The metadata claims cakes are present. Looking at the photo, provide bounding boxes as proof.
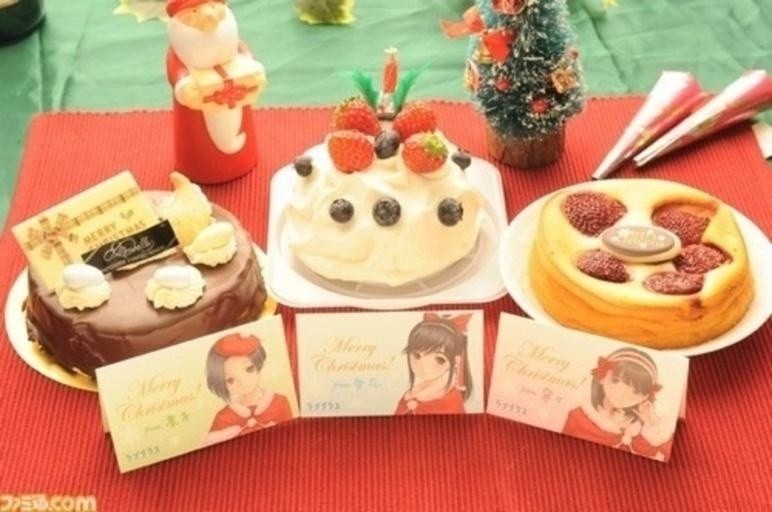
[527,177,754,350]
[286,48,485,286]
[11,171,267,383]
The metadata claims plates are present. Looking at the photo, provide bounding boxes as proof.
[264,152,511,309]
[2,239,277,394]
[497,176,771,356]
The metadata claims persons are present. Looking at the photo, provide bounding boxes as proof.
[560,345,665,459]
[392,311,475,416]
[163,1,267,185]
[205,331,294,445]
